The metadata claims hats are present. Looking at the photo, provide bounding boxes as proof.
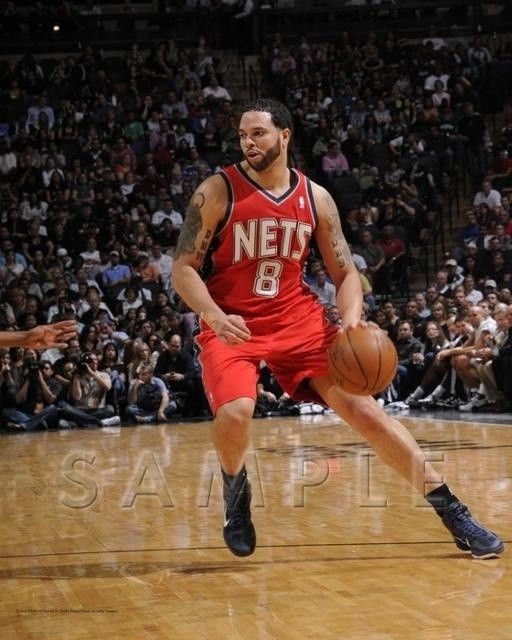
[137,251,149,258]
[110,251,119,256]
[446,260,457,266]
[485,279,497,287]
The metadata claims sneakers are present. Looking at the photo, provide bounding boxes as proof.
[135,414,147,423]
[58,419,77,427]
[39,421,48,428]
[287,404,299,415]
[405,394,510,413]
[7,422,23,431]
[101,416,121,427]
[223,489,256,556]
[443,503,504,558]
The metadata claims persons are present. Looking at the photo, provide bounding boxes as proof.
[1,2,510,435]
[169,98,504,561]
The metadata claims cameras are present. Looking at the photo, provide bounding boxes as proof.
[76,355,92,375]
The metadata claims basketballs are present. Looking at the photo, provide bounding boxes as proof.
[328,325,399,396]
